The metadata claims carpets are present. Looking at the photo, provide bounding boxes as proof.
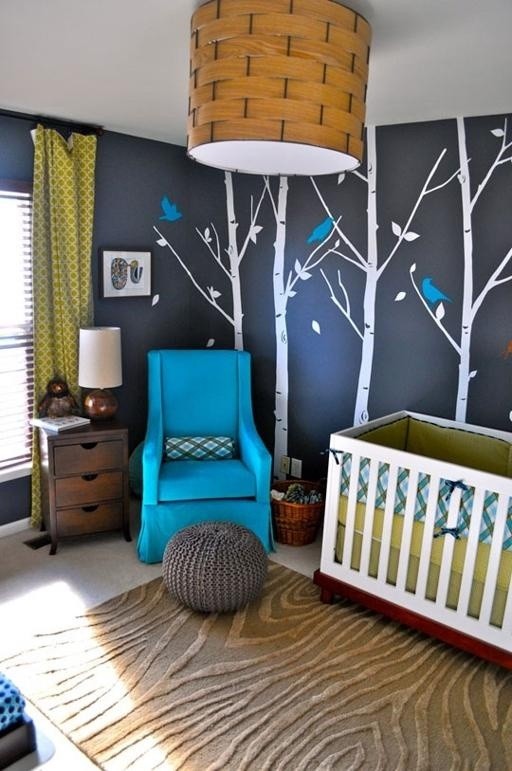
[0,558,512,770]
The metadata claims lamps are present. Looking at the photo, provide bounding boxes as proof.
[78,326,123,421]
[186,0,372,176]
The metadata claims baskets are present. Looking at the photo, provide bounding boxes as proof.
[270,480,326,548]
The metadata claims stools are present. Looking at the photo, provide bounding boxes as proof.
[162,521,269,613]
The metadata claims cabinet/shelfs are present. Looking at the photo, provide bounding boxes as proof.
[38,420,132,555]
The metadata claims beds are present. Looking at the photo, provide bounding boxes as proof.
[313,411,512,671]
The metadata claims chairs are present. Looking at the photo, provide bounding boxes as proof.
[137,349,273,564]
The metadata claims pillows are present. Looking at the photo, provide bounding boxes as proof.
[165,435,237,461]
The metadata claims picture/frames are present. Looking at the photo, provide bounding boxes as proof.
[97,248,155,301]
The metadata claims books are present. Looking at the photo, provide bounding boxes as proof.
[31,414,91,432]
